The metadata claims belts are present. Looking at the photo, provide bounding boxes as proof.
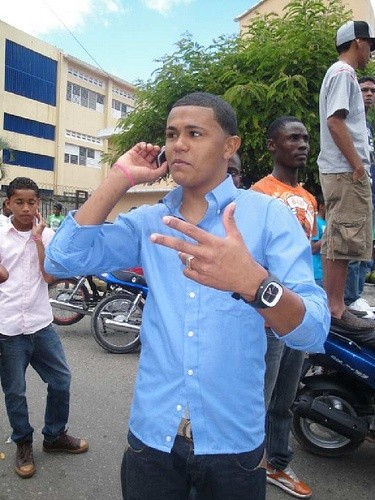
[176,418,193,441]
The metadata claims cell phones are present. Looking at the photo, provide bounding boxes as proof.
[156,149,166,168]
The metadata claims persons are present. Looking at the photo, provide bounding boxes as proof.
[43,93,331,500]
[345,77,375,321]
[320,20,375,333]
[0,199,12,228]
[246,116,318,498]
[224,154,243,187]
[47,203,65,231]
[311,195,327,287]
[0,177,89,478]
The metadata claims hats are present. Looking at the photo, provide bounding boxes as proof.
[335,21,375,52]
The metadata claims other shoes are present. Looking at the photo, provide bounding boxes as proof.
[15,442,36,477]
[331,306,375,330]
[42,436,89,453]
[266,463,313,498]
[347,299,375,320]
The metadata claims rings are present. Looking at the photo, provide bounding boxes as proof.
[186,256,197,269]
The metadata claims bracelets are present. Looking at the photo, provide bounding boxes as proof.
[31,236,42,241]
[111,162,136,187]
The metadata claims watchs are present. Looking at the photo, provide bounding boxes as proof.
[231,263,284,309]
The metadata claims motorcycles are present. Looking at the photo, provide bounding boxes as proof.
[291,321,375,458]
[48,275,136,325]
[91,267,149,353]
